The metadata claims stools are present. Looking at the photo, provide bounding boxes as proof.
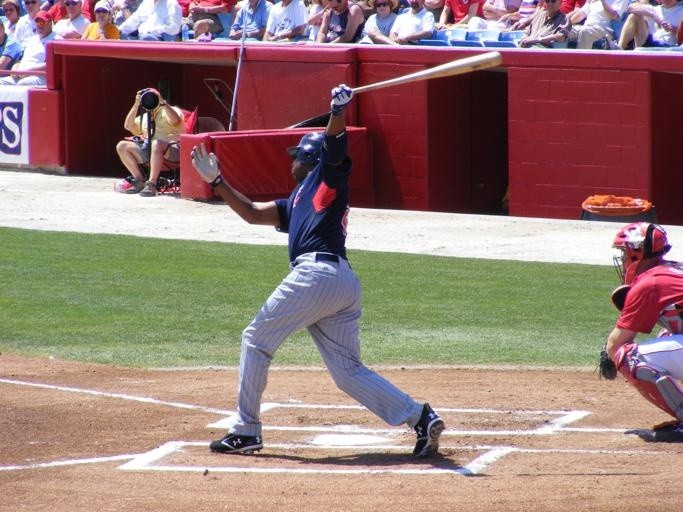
[578,194,660,224]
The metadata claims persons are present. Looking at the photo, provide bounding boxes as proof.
[1,0,683,88]
[596,219,683,440]
[115,88,185,197]
[186,82,445,460]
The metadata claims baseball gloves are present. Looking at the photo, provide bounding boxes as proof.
[611,284,631,311]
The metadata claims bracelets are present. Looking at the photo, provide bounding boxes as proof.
[160,99,168,106]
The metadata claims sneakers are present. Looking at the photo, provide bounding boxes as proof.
[140,180,156,196]
[413,403,444,458]
[653,421,683,433]
[210,435,263,454]
[126,179,143,194]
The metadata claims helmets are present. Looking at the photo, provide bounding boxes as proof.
[612,222,671,285]
[286,133,325,164]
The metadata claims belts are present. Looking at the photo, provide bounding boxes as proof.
[317,254,338,262]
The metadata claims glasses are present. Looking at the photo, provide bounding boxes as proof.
[3,0,110,26]
[375,2,389,7]
[545,0,556,3]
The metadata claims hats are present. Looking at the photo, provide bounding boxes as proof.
[366,0,399,9]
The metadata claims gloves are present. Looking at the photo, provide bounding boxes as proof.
[331,83,353,115]
[191,143,223,186]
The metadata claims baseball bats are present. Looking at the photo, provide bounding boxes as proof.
[349,52,503,93]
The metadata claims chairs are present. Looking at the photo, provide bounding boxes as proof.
[125,107,198,194]
